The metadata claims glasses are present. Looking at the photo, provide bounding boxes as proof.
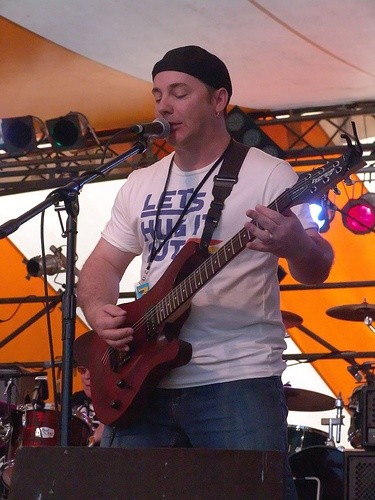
[77,366,88,374]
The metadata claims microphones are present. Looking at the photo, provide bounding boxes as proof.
[128,117,172,137]
[32,380,43,405]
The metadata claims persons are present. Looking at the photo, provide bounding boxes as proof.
[77,46,335,500]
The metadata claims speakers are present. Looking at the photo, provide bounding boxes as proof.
[8,445,300,500]
[343,386,375,500]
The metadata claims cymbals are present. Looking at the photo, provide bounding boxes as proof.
[281,310,304,329]
[326,302,375,322]
[283,386,337,412]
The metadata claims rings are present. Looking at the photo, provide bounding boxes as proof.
[263,233,272,244]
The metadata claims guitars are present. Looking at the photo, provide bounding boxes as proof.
[88,149,369,426]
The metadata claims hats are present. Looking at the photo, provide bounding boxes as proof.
[151,45,233,101]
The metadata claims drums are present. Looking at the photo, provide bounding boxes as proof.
[288,424,345,500]
[0,400,94,490]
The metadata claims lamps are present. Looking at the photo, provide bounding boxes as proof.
[0,116,46,157]
[227,105,289,159]
[342,198,375,235]
[45,112,90,151]
[309,198,335,233]
[23,255,64,280]
